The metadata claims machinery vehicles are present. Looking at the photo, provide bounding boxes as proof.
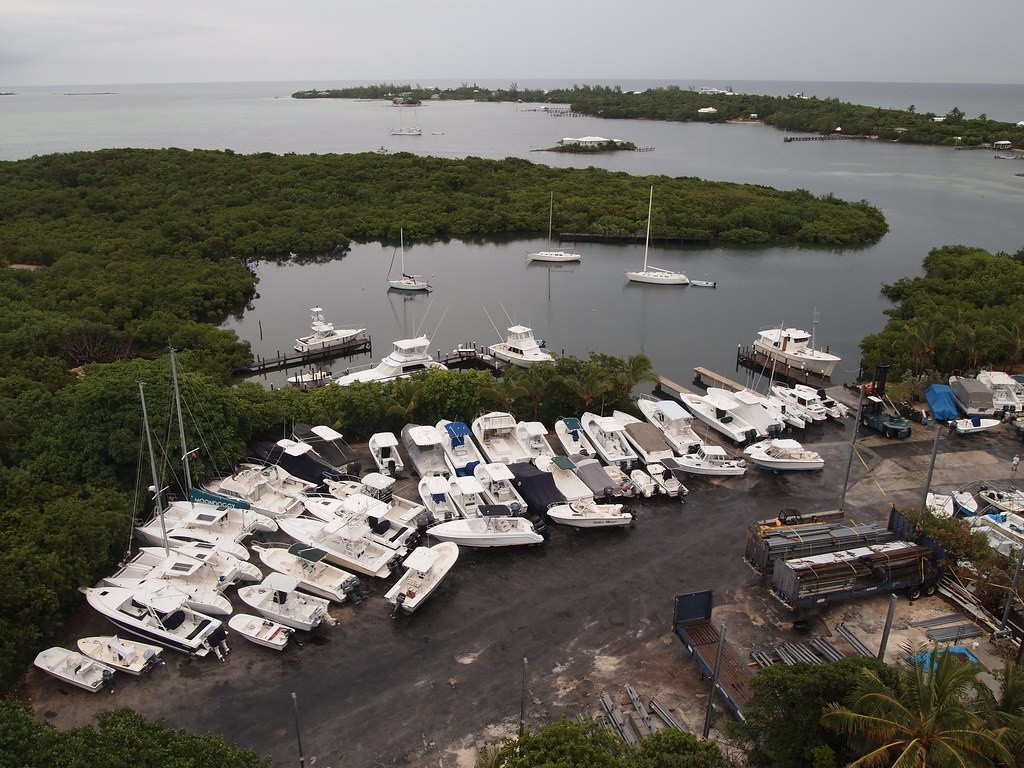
[859,363,913,441]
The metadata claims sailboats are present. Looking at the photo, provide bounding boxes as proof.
[386,227,435,291]
[626,186,691,285]
[527,191,581,262]
[388,106,423,135]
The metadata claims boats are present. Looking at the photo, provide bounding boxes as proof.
[331,298,447,391]
[286,370,331,392]
[483,301,555,370]
[926,368,1024,435]
[37,339,868,700]
[927,471,1024,576]
[691,280,716,287]
[754,307,841,378]
[294,305,367,352]
